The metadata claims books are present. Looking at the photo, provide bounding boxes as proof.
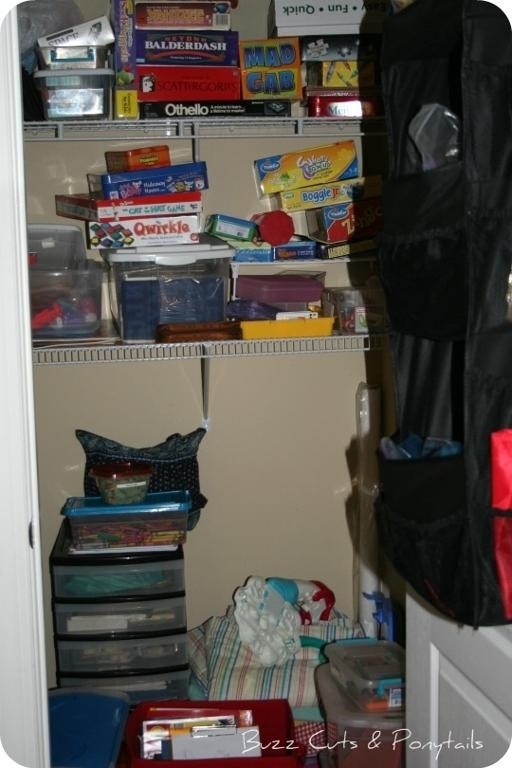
[139,705,261,762]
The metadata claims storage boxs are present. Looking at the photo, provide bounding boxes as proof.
[51,490,401,762]
[32,68,116,121]
[25,224,236,341]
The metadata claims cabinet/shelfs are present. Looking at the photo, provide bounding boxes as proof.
[23,115,367,421]
[405,591,512,768]
